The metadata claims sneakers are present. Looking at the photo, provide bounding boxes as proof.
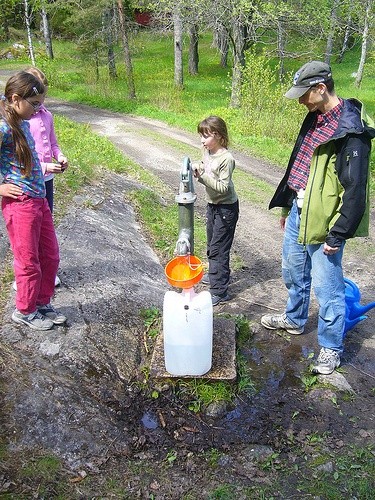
[261,312,304,335]
[202,274,231,284]
[312,347,341,374]
[11,308,53,330]
[36,304,67,324]
[212,292,229,305]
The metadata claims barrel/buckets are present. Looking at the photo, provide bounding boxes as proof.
[163,287,213,377]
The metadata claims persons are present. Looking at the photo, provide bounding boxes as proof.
[260,61,374,374]
[191,116,239,306]
[0,71,66,331]
[12,66,68,291]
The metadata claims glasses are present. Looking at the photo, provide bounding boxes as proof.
[10,94,41,112]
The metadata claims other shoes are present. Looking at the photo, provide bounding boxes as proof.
[55,276,61,287]
[13,281,17,290]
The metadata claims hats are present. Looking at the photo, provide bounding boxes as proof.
[284,61,332,100]
